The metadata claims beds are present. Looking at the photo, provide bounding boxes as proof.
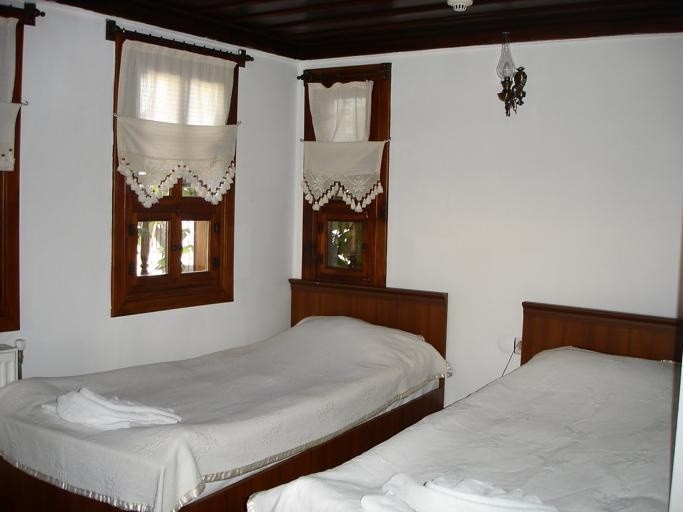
[0,273,453,512]
[245,299,680,512]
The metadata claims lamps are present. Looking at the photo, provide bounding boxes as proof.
[492,28,529,118]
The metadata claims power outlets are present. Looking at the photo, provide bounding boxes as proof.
[512,337,524,356]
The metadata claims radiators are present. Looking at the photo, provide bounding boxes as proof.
[0,335,25,387]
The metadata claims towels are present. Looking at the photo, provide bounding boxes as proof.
[359,469,560,512]
[36,387,183,434]
